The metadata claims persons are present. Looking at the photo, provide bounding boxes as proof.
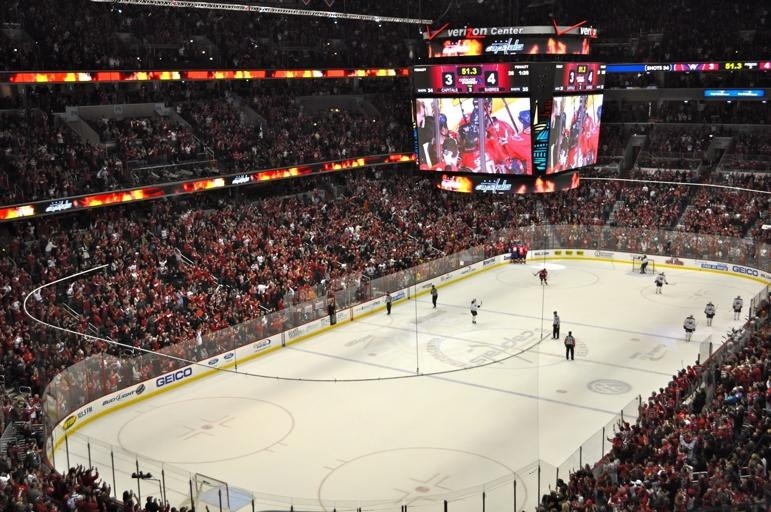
[598,71,771,188]
[416,97,532,174]
[410,169,771,279]
[430,271,770,511]
[547,95,602,174]
[1,3,412,512]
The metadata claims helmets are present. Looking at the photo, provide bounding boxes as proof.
[438,97,532,158]
[560,95,602,156]
